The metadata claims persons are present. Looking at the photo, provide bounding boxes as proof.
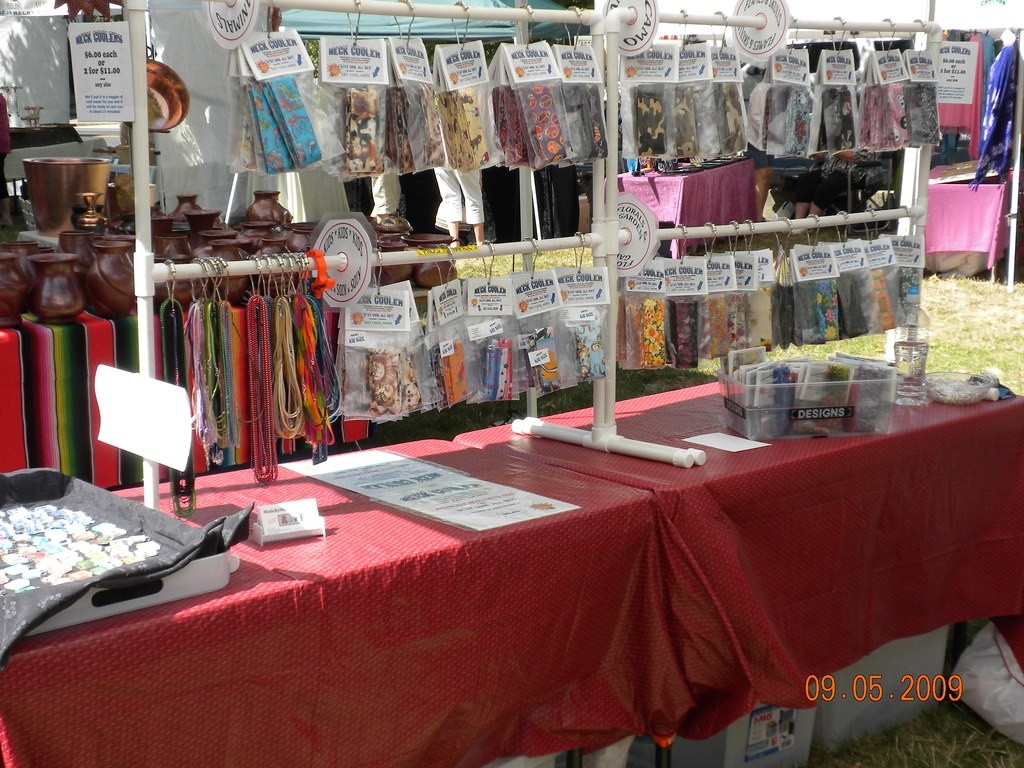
[434,167,485,248]
[789,172,843,236]
[747,69,773,223]
[371,173,401,218]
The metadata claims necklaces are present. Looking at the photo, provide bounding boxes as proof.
[185,295,342,487]
[160,297,195,522]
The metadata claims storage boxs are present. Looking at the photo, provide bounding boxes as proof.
[628,696,816,768]
[807,625,947,749]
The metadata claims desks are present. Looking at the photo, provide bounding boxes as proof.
[923,165,1020,284]
[0,379,1024,768]
[611,158,758,259]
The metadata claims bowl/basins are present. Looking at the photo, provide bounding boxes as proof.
[925,372,997,404]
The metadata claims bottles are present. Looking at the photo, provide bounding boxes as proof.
[115,212,135,234]
[75,193,108,232]
[371,223,458,288]
[0,231,136,327]
[151,190,315,306]
[894,287,929,405]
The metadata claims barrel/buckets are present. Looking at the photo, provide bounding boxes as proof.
[23,158,111,236]
